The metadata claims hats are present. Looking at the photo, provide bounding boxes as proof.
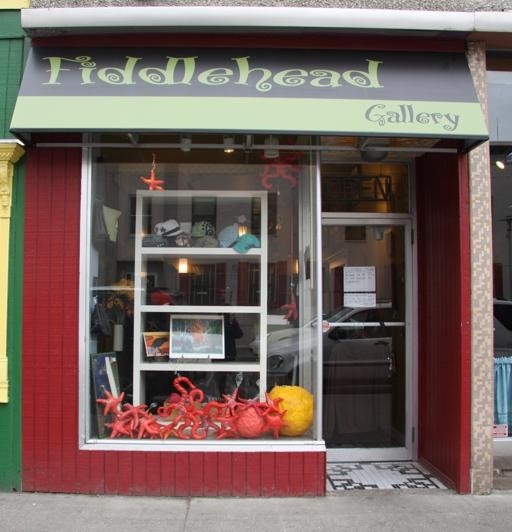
[143,219,261,253]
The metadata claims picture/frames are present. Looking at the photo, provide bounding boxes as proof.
[169,314,225,359]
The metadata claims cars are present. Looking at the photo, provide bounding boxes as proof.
[249,302,512,394]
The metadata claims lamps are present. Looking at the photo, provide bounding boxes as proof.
[224,138,235,154]
[181,138,192,153]
[264,136,280,158]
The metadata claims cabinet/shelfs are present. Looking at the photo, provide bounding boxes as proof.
[132,191,268,432]
